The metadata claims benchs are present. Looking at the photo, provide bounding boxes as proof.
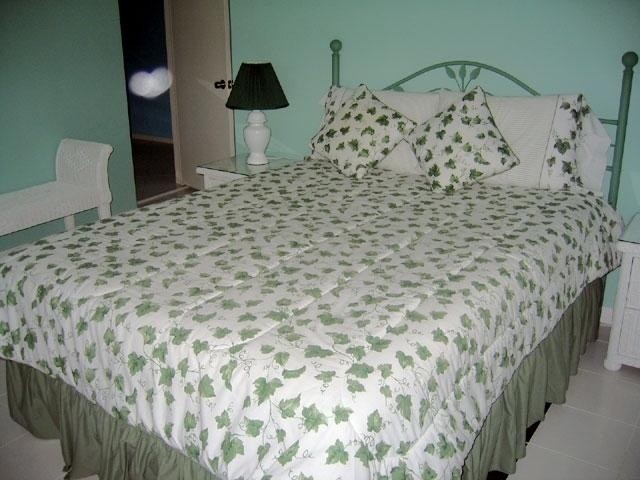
[1,137,115,235]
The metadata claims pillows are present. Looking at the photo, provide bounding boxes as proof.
[307,84,589,195]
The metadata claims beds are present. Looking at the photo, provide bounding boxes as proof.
[0,41,640,480]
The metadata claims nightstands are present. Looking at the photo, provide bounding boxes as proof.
[195,155,296,188]
[603,213,640,371]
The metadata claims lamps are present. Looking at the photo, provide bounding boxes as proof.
[225,61,290,164]
